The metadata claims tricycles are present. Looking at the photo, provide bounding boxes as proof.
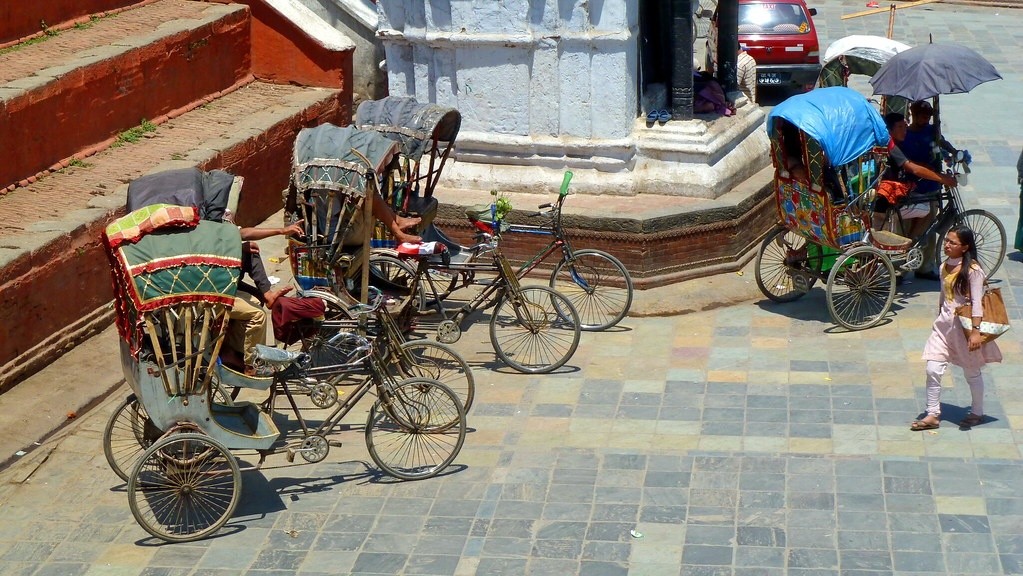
[753,86,1009,333]
[100,94,635,546]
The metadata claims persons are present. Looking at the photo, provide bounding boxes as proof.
[737,42,757,102]
[195,220,304,376]
[312,186,423,244]
[880,101,965,288]
[911,225,1003,431]
[1015,149,1023,256]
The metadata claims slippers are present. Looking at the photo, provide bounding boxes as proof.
[251,367,275,377]
[912,419,939,431]
[646,109,672,122]
[960,416,983,427]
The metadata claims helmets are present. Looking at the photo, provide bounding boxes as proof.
[911,101,932,116]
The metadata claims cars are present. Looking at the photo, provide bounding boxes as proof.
[704,0,823,94]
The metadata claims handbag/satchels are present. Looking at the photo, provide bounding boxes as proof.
[955,261,1011,348]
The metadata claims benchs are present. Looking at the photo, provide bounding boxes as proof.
[288,230,370,308]
[119,330,223,437]
[777,175,912,253]
[371,161,438,262]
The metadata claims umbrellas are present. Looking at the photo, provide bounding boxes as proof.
[868,32,1005,102]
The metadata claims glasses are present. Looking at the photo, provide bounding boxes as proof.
[944,238,962,248]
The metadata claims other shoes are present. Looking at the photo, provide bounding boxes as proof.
[896,278,912,285]
[914,271,938,281]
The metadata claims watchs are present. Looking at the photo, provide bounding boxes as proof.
[972,325,980,330]
